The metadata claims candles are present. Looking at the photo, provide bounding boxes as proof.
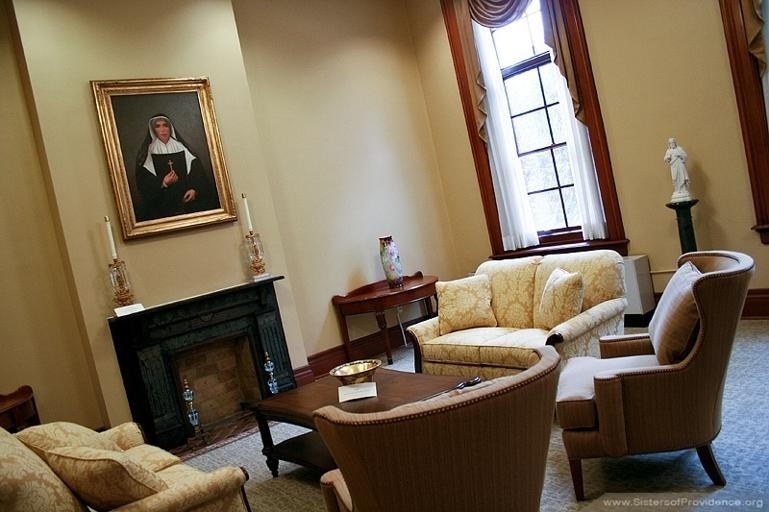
[103,217,118,260]
[242,197,253,235]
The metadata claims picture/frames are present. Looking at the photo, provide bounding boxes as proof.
[88,76,239,242]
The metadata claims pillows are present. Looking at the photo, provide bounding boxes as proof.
[538,268,583,332]
[433,275,498,332]
[44,447,168,512]
[648,263,703,365]
[13,422,121,459]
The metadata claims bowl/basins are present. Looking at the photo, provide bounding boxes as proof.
[330,358,382,385]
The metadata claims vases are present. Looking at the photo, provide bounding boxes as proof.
[378,235,403,289]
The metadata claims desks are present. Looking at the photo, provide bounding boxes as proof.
[330,271,439,366]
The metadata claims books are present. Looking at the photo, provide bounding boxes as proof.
[338,381,377,403]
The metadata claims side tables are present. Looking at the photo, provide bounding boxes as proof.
[0,385,43,435]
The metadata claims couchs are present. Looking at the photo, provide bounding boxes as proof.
[0,423,251,512]
[555,249,754,503]
[406,251,624,383]
[312,345,562,512]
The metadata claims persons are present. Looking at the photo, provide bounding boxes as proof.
[135,112,221,221]
[664,138,693,203]
